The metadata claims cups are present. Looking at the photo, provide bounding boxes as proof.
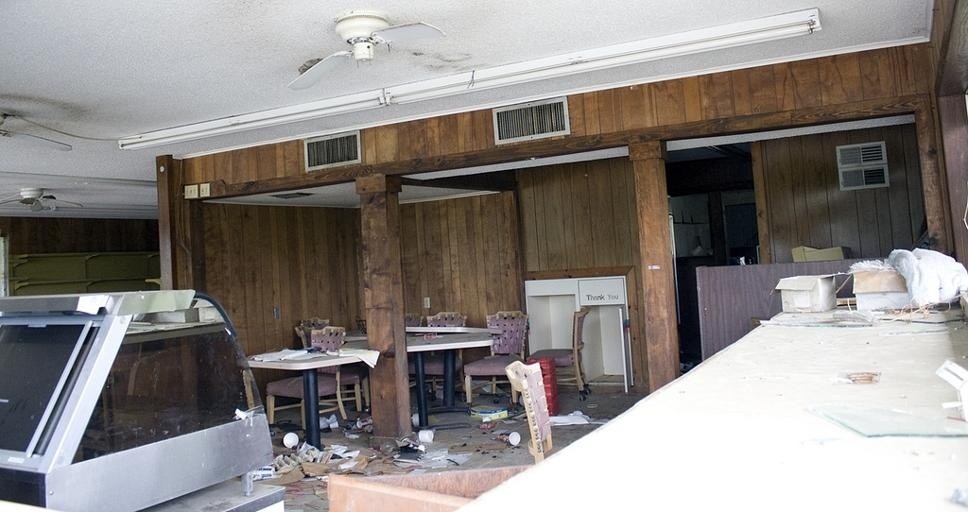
[356,416,373,429]
[278,431,300,447]
[411,413,420,428]
[419,430,434,443]
[497,430,521,446]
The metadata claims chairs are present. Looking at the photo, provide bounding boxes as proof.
[464,311,528,416]
[245,312,503,452]
[530,306,591,401]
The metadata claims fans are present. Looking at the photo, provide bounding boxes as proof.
[0,184,85,212]
[1,118,72,154]
[286,11,476,95]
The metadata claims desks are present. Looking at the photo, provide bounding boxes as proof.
[450,302,967,512]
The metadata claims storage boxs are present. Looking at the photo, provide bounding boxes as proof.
[769,271,849,314]
[851,272,934,312]
[792,246,844,263]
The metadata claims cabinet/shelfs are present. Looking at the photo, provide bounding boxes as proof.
[522,266,635,395]
[1,293,288,511]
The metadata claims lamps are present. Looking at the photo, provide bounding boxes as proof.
[381,7,824,109]
[114,79,390,153]
